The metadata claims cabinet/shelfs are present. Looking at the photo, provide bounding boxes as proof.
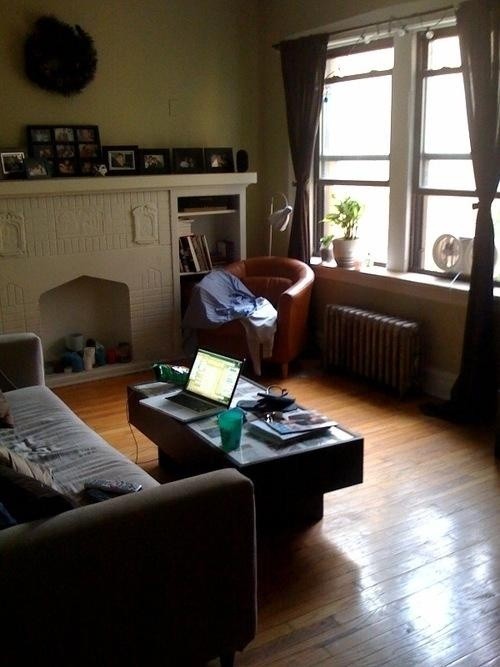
[178,206,238,276]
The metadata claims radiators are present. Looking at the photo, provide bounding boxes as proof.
[319,301,422,403]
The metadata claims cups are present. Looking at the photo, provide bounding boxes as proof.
[65,332,84,350]
[95,347,129,365]
[64,367,73,376]
[217,408,244,450]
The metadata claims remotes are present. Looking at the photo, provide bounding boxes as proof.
[84,478,143,493]
[83,489,113,503]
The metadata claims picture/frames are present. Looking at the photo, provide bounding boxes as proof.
[0,123,235,179]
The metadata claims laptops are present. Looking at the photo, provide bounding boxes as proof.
[137,346,247,424]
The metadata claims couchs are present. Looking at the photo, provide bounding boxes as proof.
[0,332,259,665]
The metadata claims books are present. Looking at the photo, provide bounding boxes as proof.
[177,198,236,274]
[247,408,339,446]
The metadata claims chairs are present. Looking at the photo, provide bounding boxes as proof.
[187,254,315,378]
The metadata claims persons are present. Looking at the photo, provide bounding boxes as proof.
[29,162,46,175]
[144,156,162,169]
[78,129,93,142]
[113,153,133,167]
[180,157,195,168]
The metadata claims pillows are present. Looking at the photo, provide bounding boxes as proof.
[1,389,14,429]
[1,465,64,521]
[0,440,64,504]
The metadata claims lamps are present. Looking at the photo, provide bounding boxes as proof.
[268,190,293,256]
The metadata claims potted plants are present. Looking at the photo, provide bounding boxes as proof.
[317,192,361,268]
